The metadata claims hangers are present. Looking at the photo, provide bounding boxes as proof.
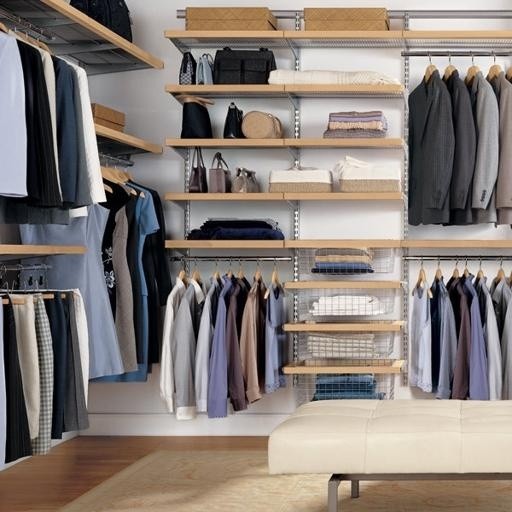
[93,156,139,200]
[420,47,511,87]
[173,254,287,301]
[0,11,59,62]
[410,254,511,297]
[0,265,80,312]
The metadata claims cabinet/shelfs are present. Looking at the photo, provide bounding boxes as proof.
[282,277,408,376]
[0,0,165,258]
[163,10,512,261]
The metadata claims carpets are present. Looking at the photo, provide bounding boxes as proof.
[57,450,511,512]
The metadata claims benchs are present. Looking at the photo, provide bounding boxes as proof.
[268,399,512,512]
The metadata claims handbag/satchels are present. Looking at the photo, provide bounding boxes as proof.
[196,54,214,86]
[179,52,197,84]
[209,152,231,193]
[224,103,243,138]
[189,146,207,193]
[241,111,284,139]
[231,168,259,193]
[215,48,277,84]
